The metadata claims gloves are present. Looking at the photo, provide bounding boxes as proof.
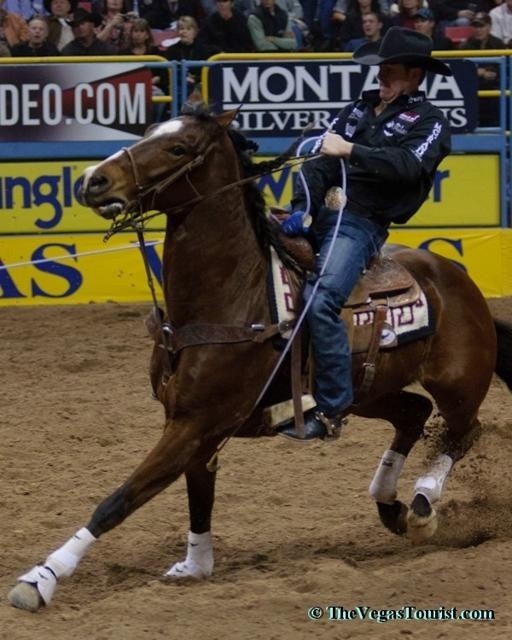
[282,211,312,236]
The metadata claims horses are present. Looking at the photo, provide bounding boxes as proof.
[6,82,512,613]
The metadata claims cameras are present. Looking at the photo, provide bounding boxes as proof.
[120,14,129,23]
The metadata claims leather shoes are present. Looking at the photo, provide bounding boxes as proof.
[281,410,342,442]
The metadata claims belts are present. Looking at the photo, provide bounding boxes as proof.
[323,186,347,210]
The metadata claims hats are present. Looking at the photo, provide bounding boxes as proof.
[471,13,490,24]
[412,8,434,19]
[352,27,452,75]
[65,8,102,27]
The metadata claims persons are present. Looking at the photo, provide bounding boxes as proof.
[0,0,511,136]
[281,28,452,442]
